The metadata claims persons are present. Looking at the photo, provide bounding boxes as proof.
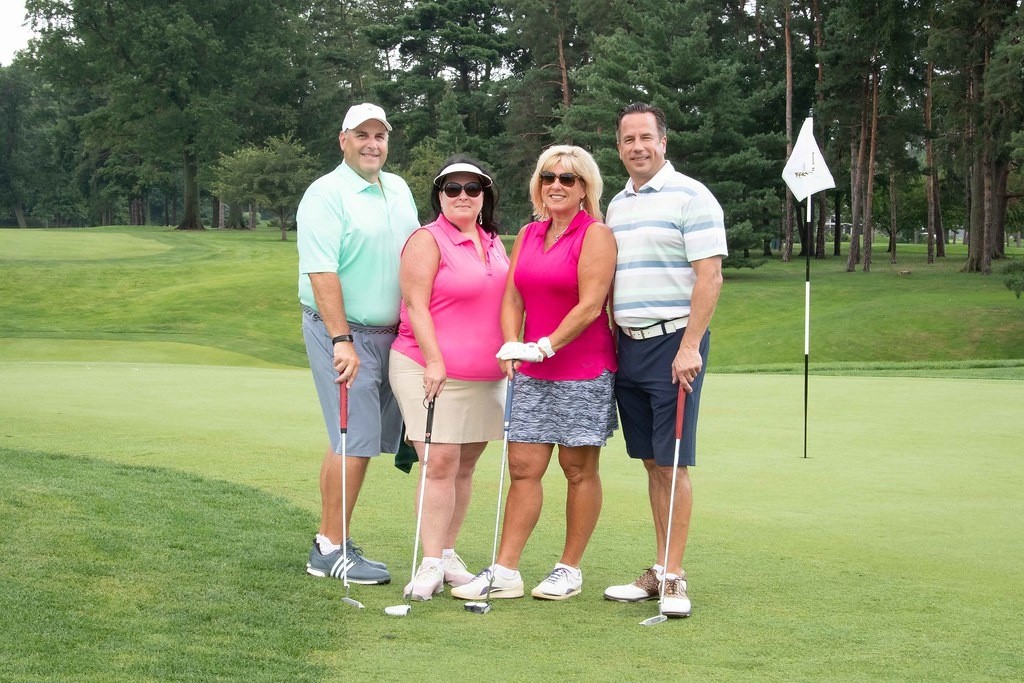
[388,159,510,605]
[295,102,421,584]
[450,144,618,600]
[605,102,730,617]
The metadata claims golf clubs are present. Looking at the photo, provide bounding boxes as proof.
[340,370,365,609]
[638,382,686,626]
[384,393,436,617]
[463,356,516,615]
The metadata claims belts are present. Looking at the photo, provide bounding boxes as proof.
[621,316,689,341]
[301,303,399,335]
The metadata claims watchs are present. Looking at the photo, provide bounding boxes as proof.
[331,334,354,345]
[537,336,555,358]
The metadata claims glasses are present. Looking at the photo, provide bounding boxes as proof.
[439,182,485,199]
[538,171,580,187]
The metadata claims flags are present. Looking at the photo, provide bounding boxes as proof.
[781,117,835,202]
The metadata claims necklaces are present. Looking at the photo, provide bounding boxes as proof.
[552,225,568,242]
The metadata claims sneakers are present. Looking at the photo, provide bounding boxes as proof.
[658,575,692,618]
[403,562,445,601]
[450,566,525,601]
[531,563,582,601]
[603,563,665,602]
[307,539,391,585]
[306,538,387,570]
[443,558,477,587]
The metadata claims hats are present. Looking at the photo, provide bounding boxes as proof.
[342,102,392,133]
[433,162,492,189]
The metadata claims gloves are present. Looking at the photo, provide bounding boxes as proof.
[496,342,543,363]
